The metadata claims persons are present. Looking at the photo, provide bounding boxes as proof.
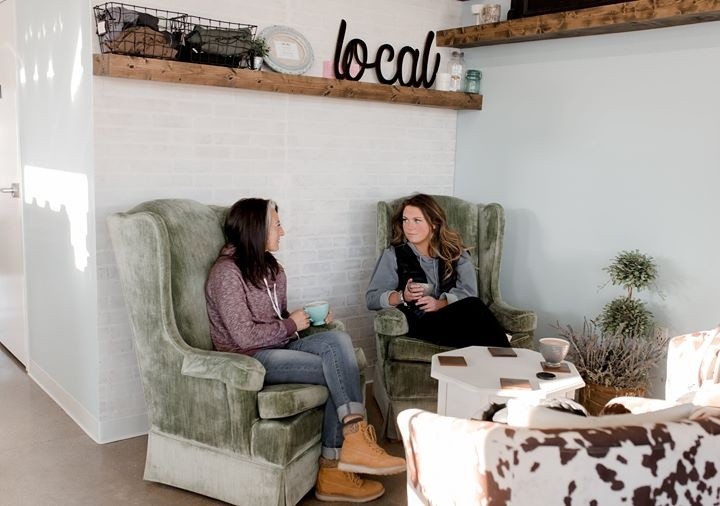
[364,194,512,348]
[204,197,406,502]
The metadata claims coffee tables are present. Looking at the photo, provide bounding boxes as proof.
[431,346,586,422]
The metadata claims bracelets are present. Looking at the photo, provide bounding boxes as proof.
[400,290,408,306]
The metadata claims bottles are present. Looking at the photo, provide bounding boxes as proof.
[449,50,466,91]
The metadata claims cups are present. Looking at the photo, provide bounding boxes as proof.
[480,3,501,23]
[464,69,482,94]
[303,301,329,326]
[436,73,458,92]
[539,337,570,368]
[412,282,434,296]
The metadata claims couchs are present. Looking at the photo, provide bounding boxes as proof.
[398,326,720,506]
[103,196,367,506]
[374,192,539,441]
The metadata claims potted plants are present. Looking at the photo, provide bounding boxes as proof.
[560,247,669,416]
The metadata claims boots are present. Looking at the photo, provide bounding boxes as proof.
[338,422,407,476]
[315,458,385,503]
